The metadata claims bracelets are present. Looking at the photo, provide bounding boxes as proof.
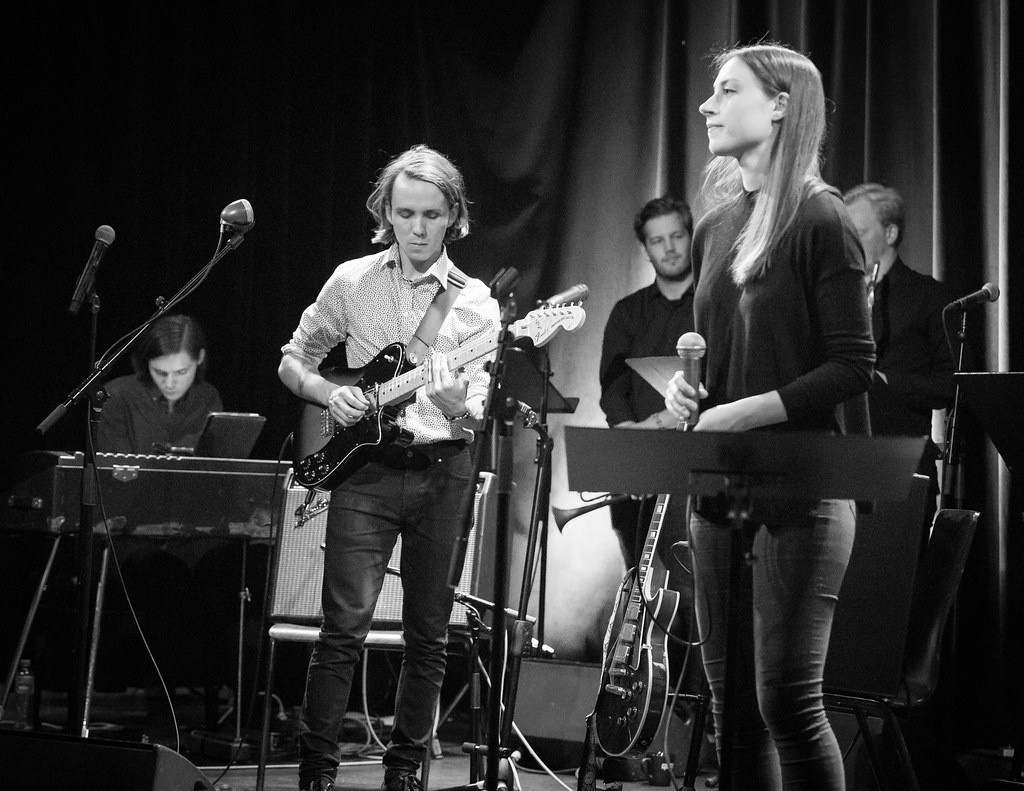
[650,412,665,429]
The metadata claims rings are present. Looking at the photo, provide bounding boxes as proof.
[347,415,353,419]
[427,380,434,383]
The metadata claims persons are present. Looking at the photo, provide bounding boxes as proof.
[276,143,502,791]
[91,313,228,761]
[600,193,694,731]
[685,45,965,791]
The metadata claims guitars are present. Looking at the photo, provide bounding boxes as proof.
[290,300,587,492]
[594,417,680,759]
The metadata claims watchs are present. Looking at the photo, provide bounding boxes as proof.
[442,411,471,423]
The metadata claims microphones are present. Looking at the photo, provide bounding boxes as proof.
[944,282,999,314]
[549,284,590,308]
[218,199,254,235]
[70,225,115,315]
[675,331,707,431]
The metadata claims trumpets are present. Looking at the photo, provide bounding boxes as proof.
[551,494,634,534]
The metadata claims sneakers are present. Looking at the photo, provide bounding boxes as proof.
[299,777,333,791]
[381,768,423,791]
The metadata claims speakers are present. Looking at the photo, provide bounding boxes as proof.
[509,655,712,779]
[0,730,216,791]
[820,472,934,696]
[270,467,497,634]
